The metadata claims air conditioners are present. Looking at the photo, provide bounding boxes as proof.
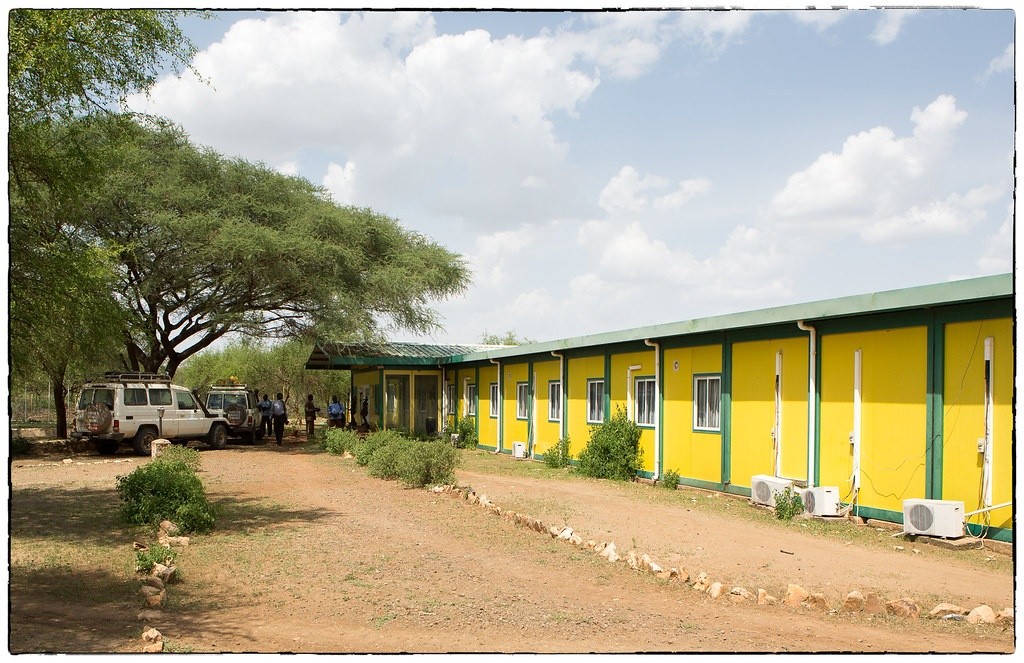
[511,442,527,458]
[803,486,841,517]
[902,498,966,539]
[752,474,795,510]
[450,433,459,447]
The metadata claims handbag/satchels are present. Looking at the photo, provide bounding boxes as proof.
[342,413,345,427]
[284,414,287,423]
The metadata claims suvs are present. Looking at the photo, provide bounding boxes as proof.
[206,385,267,444]
[70,371,231,455]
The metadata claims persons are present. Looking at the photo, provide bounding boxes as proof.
[360,395,369,425]
[257,393,289,446]
[304,394,321,439]
[328,395,345,432]
[348,396,357,423]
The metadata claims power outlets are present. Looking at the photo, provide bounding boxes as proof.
[771,428,776,438]
[849,432,854,443]
[978,438,984,453]
